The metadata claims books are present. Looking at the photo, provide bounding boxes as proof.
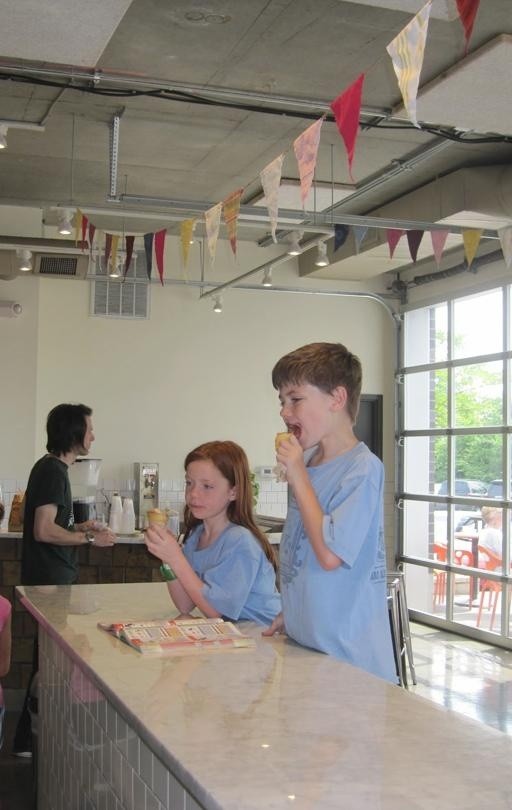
[100,615,258,658]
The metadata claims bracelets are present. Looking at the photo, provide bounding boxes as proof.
[159,563,178,584]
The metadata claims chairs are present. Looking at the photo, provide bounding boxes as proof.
[433,545,503,631]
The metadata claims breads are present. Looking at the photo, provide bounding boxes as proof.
[274,432,292,452]
[146,509,167,533]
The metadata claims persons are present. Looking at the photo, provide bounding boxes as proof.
[259,340,400,687]
[12,401,117,758]
[479,505,503,576]
[143,439,282,625]
[0,592,12,751]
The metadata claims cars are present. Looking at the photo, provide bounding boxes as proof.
[433,477,512,591]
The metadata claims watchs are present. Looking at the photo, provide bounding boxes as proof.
[86,531,97,546]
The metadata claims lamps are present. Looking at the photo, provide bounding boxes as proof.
[198,227,335,312]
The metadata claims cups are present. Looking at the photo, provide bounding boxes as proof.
[108,495,136,534]
